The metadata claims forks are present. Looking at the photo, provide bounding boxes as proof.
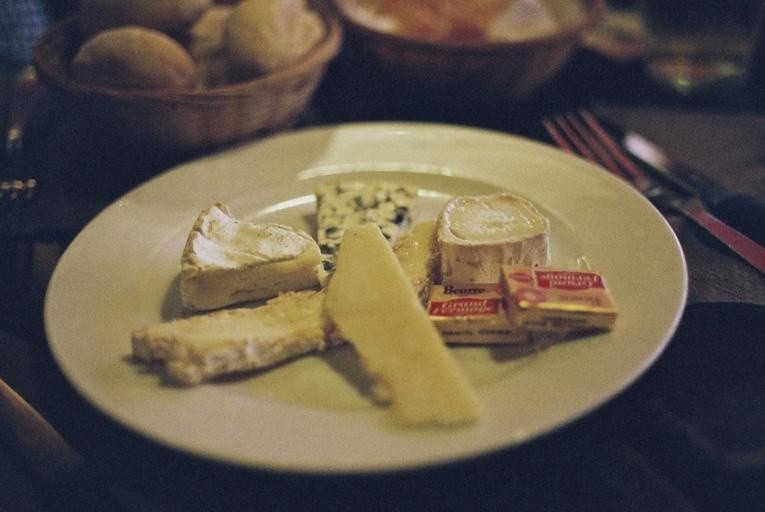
[0,64,55,208]
[541,105,760,275]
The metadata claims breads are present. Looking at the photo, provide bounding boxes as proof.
[132,180,549,433]
[69,1,328,92]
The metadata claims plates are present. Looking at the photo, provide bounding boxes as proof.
[41,122,693,482]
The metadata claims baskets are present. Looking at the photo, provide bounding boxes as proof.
[31,4,344,151]
